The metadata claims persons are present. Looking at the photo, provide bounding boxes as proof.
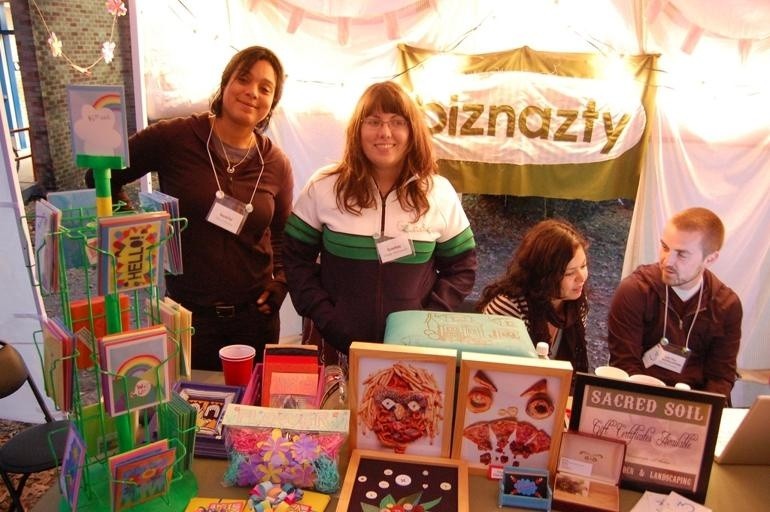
[477,217,590,381]
[81,45,297,373]
[279,84,480,369]
[604,204,746,414]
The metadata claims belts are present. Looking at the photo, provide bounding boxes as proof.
[187,303,256,318]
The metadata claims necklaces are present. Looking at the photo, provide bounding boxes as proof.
[213,116,255,175]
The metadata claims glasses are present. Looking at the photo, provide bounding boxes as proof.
[361,118,407,129]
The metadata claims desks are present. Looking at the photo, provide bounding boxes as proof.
[32,367,770,511]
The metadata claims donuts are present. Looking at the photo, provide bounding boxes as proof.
[526,398,555,419]
[468,390,492,414]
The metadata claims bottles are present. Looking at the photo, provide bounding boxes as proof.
[534,341,550,362]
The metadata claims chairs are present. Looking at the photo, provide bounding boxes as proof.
[1,339,70,512]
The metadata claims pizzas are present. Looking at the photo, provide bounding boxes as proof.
[462,420,551,457]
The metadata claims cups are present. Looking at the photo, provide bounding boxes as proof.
[218,344,256,386]
[595,364,667,390]
